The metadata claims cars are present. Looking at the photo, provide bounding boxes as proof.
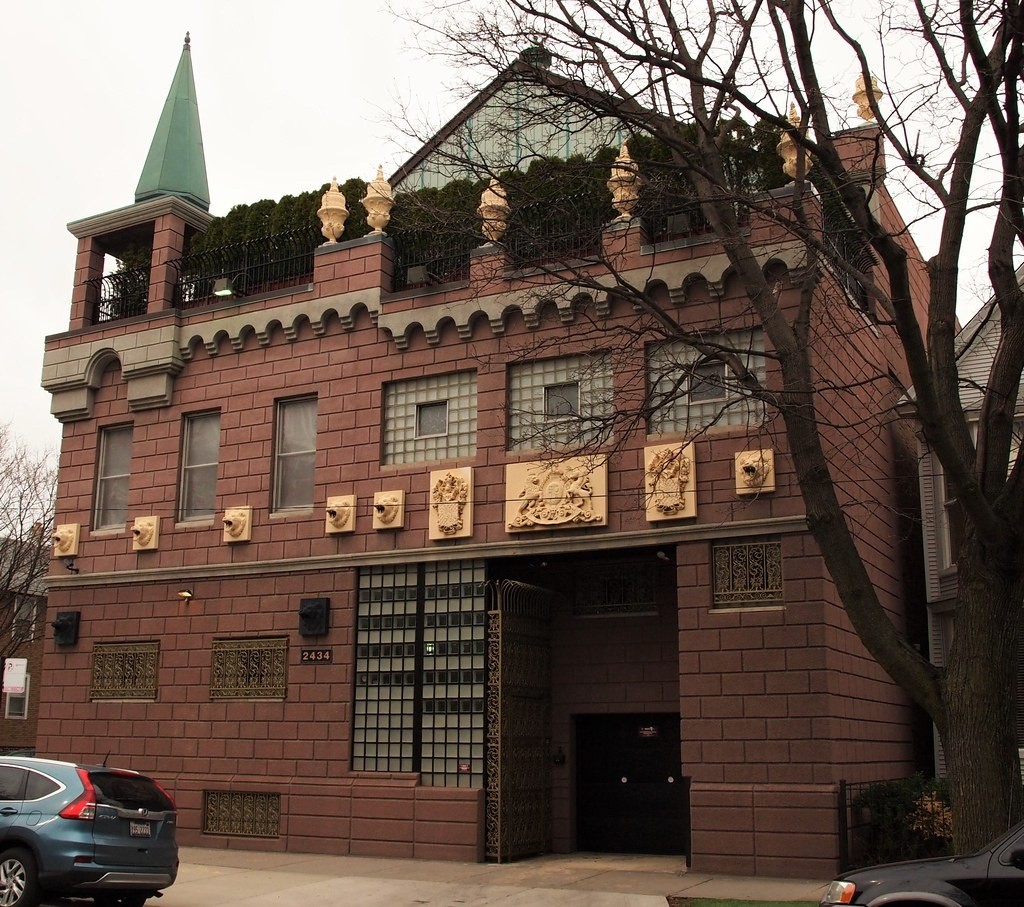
[819,819,1024,907]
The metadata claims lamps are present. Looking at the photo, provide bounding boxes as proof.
[554,748,564,767]
[177,590,193,605]
[212,273,251,297]
[406,266,444,286]
[63,558,79,573]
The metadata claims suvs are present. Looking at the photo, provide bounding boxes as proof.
[0,756,180,907]
